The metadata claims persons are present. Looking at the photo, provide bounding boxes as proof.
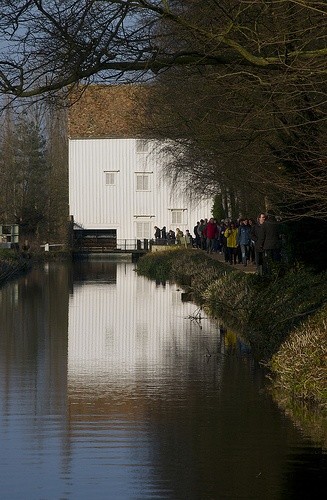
[21,240,30,252]
[154,210,285,275]
